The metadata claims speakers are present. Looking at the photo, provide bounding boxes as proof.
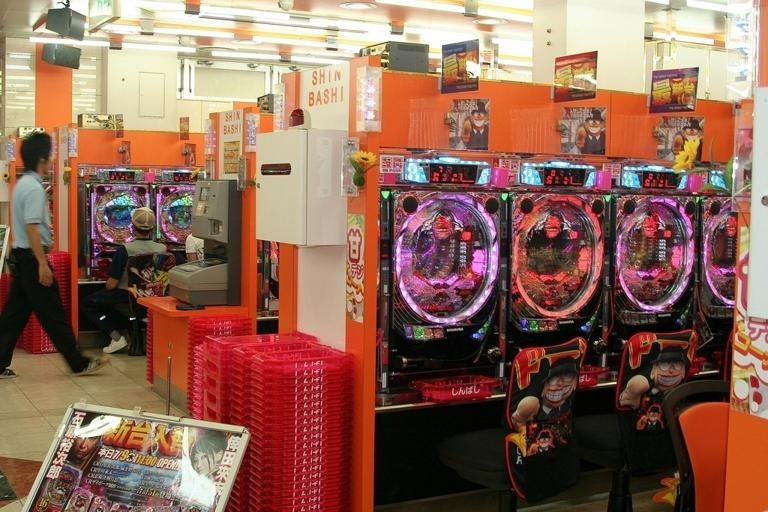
[46,8,86,41]
[41,44,81,70]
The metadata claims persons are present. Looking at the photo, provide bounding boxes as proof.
[0,131,111,379]
[189,430,228,476]
[73,436,100,461]
[80,206,168,354]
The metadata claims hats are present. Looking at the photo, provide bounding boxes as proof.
[130,207,155,231]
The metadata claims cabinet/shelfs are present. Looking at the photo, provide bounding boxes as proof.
[135,296,249,417]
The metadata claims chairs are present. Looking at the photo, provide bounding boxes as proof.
[444,335,590,511]
[662,377,727,511]
[115,252,176,356]
[572,327,700,510]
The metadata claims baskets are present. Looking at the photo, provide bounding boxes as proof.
[186,311,346,511]
[578,365,608,389]
[1,251,71,355]
[414,374,499,404]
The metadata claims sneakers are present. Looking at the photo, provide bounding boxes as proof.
[102,335,128,354]
[0,366,17,379]
[71,356,110,376]
